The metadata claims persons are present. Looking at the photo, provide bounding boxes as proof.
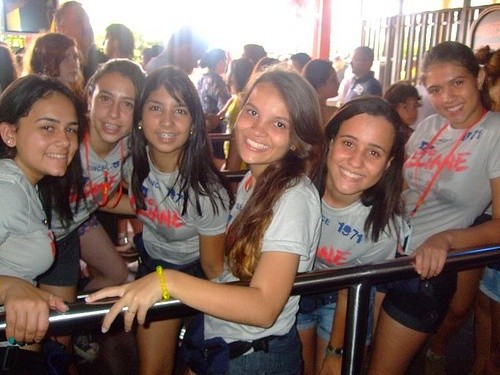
[367,42,499,375]
[121,64,229,375]
[295,97,404,375]
[3,58,147,375]
[0,1,500,375]
[85,69,327,375]
[0,75,85,346]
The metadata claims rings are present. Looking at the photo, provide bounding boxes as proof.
[128,310,136,314]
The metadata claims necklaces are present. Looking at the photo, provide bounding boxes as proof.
[45,220,47,224]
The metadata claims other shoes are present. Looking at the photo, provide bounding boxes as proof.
[73,336,96,359]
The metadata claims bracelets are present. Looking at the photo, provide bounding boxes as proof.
[156,266,170,301]
[328,342,343,354]
